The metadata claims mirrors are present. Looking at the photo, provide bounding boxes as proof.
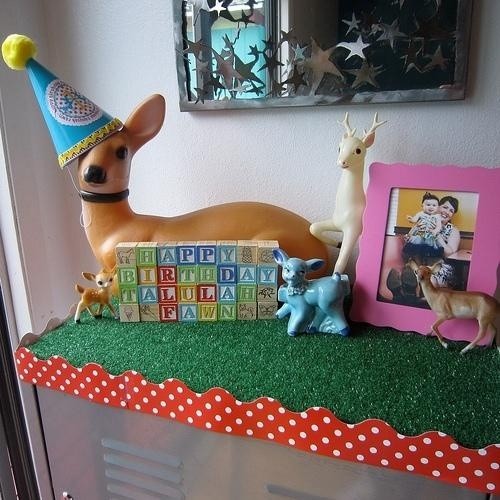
[172,0,473,112]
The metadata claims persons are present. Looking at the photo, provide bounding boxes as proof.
[403,191,443,266]
[404,196,461,299]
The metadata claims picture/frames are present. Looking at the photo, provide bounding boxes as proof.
[348,162,500,346]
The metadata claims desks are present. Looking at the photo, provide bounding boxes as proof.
[446,249,472,291]
